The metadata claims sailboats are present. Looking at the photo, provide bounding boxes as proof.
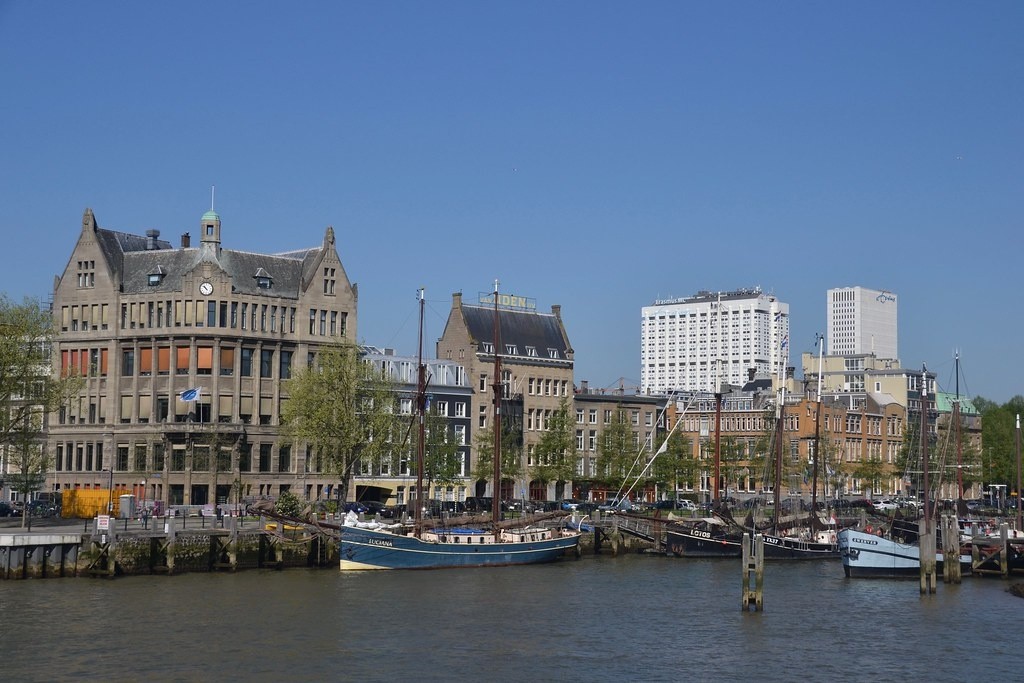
[339,278,583,571]
[744,333,840,561]
[836,363,972,580]
[606,291,752,558]
[888,347,984,548]
[970,413,1024,572]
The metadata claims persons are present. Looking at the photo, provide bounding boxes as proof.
[140,508,147,528]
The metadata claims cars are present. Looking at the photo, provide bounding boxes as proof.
[965,501,978,509]
[28,500,61,518]
[851,499,874,510]
[830,499,850,508]
[649,496,805,511]
[320,494,597,524]
[873,500,900,511]
[598,498,620,510]
[892,497,924,509]
[806,501,830,511]
[8,501,28,512]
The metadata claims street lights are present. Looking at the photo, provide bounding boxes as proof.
[101,467,113,515]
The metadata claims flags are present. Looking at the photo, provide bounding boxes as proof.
[179,386,201,401]
[774,311,781,322]
[782,336,786,348]
